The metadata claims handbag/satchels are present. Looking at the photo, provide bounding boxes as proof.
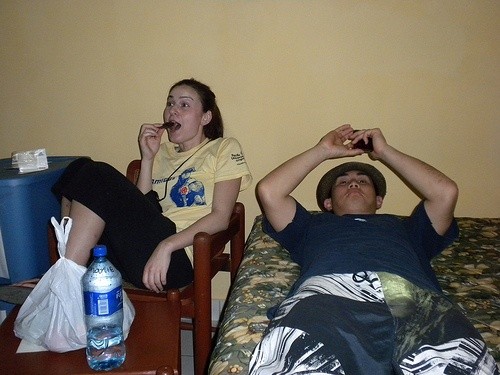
[12,216,136,353]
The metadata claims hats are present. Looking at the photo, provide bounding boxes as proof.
[316,162,387,215]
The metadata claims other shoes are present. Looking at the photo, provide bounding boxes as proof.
[0,279,41,305]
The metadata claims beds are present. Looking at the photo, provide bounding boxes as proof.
[204,212,500,375]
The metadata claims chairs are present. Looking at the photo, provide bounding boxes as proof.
[45,159,247,375]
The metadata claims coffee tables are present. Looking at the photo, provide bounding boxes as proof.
[0,289,181,375]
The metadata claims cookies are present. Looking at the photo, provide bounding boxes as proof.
[160,121,175,128]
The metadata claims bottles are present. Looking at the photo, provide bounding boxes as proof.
[80,246,128,371]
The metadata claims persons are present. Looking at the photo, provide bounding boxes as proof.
[0,76,252,305]
[246,124,499,375]
